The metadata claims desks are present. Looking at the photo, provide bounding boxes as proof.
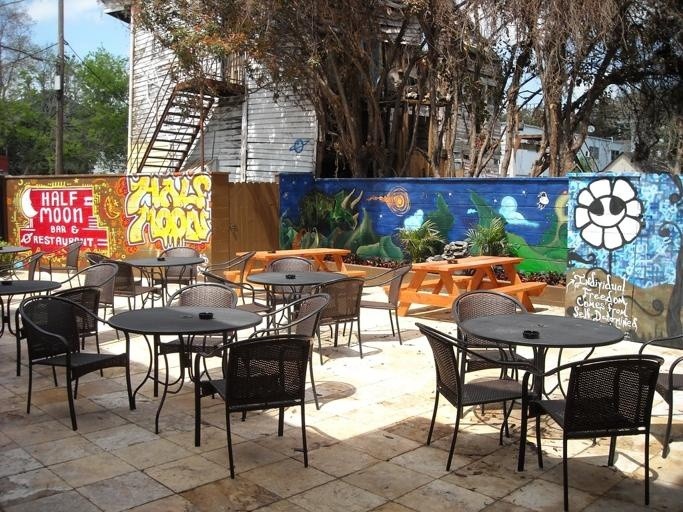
[456,314,624,446]
[396,255,524,317]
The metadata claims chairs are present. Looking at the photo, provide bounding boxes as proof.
[453,290,529,418]
[415,322,543,471]
[518,354,665,512]
[635,334,683,458]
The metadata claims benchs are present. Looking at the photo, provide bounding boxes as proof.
[384,276,472,317]
[488,282,547,313]
